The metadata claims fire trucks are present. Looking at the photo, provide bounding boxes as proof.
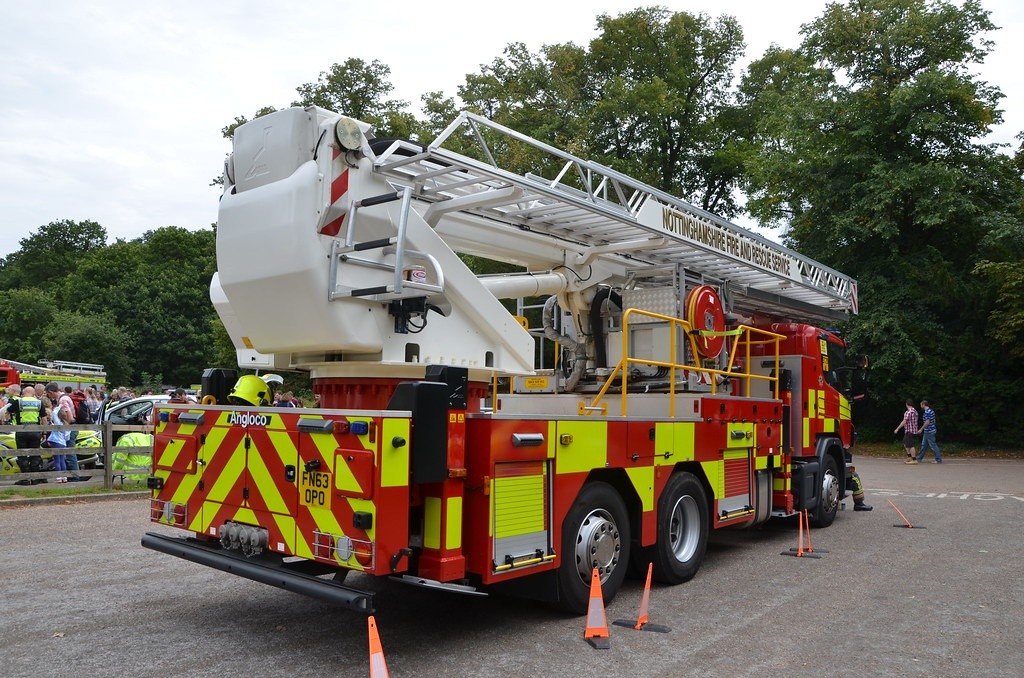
[141,98,860,623]
[0,358,106,393]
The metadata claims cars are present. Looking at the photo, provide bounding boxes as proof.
[92,392,201,466]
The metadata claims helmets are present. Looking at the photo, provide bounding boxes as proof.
[226,374,273,407]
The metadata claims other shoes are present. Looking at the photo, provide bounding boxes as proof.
[67,476,81,483]
[930,459,942,464]
[854,503,872,511]
[916,457,922,463]
[54,476,68,484]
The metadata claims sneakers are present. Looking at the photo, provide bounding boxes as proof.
[903,457,918,464]
[16,479,40,486]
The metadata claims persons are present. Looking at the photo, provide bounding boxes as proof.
[43,387,47,397]
[272,390,284,407]
[1,389,6,396]
[52,382,59,387]
[80,389,82,392]
[841,471,873,511]
[63,386,73,396]
[84,386,94,404]
[146,388,153,395]
[96,392,106,410]
[84,390,96,418]
[288,391,298,407]
[45,382,79,483]
[297,399,307,408]
[273,392,284,407]
[176,388,195,405]
[7,386,46,485]
[161,387,169,395]
[894,399,919,465]
[289,395,296,408]
[0,388,12,435]
[46,402,72,483]
[101,382,139,426]
[0,400,15,426]
[275,392,294,408]
[34,383,56,471]
[6,384,24,426]
[4,387,12,401]
[178,390,189,404]
[46,392,58,406]
[139,389,149,397]
[195,390,204,404]
[59,389,63,393]
[915,400,942,465]
[314,392,322,407]
[89,384,98,395]
[83,386,99,407]
[42,407,55,448]
